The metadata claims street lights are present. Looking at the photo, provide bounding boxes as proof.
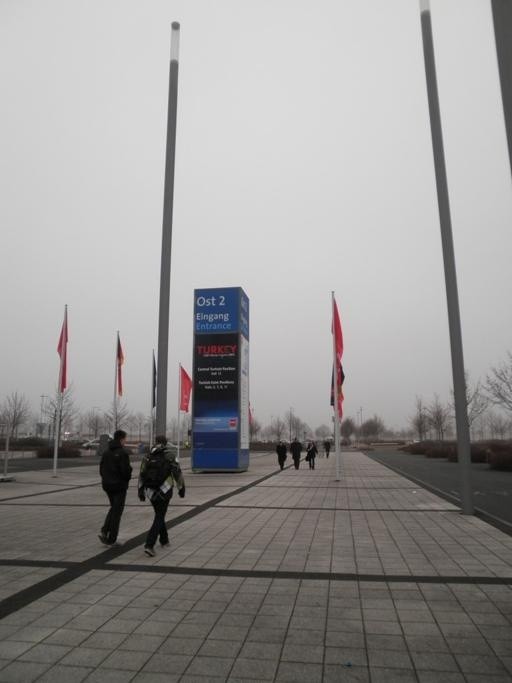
[419,0,475,516]
[157,21,180,435]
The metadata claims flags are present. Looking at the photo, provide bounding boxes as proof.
[248,400,252,425]
[179,367,195,411]
[153,353,156,409]
[56,307,69,392]
[116,334,126,397]
[330,296,345,418]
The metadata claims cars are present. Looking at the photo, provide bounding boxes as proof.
[155,440,178,450]
[81,434,115,449]
[258,435,333,443]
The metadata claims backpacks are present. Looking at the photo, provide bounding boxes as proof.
[141,449,171,489]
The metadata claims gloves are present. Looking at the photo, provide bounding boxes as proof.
[179,487,185,497]
[138,487,146,501]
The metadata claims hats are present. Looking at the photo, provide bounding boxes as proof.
[156,435,167,444]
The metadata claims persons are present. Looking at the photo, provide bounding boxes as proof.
[136,435,186,557]
[96,429,133,545]
[290,438,301,470]
[275,441,287,469]
[324,440,330,458]
[304,440,319,469]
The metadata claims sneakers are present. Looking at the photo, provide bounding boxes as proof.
[144,544,155,556]
[97,532,108,544]
[107,542,119,548]
